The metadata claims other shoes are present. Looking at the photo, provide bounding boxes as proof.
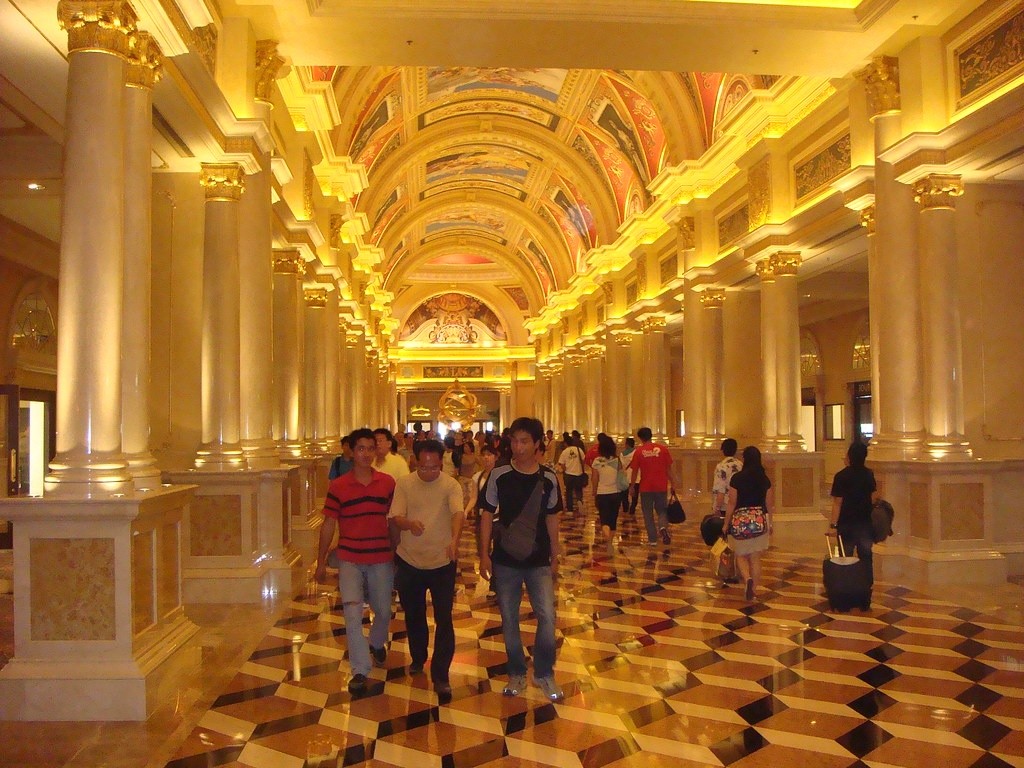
[745,577,754,603]
[370,644,385,663]
[349,674,369,692]
[503,674,526,697]
[565,511,573,517]
[660,527,671,545]
[530,674,564,702]
[577,500,584,512]
[434,676,451,696]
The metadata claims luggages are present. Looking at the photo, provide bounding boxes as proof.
[823,530,870,609]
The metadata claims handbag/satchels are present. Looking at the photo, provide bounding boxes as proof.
[699,513,726,547]
[615,457,629,490]
[581,473,588,488]
[710,533,739,584]
[730,503,767,538]
[666,494,686,524]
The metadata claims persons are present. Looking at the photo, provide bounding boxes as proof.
[315,417,586,700]
[713,439,773,603]
[825,441,879,606]
[584,427,676,546]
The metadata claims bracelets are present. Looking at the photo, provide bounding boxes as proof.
[830,524,838,529]
[769,524,773,528]
[714,505,721,511]
